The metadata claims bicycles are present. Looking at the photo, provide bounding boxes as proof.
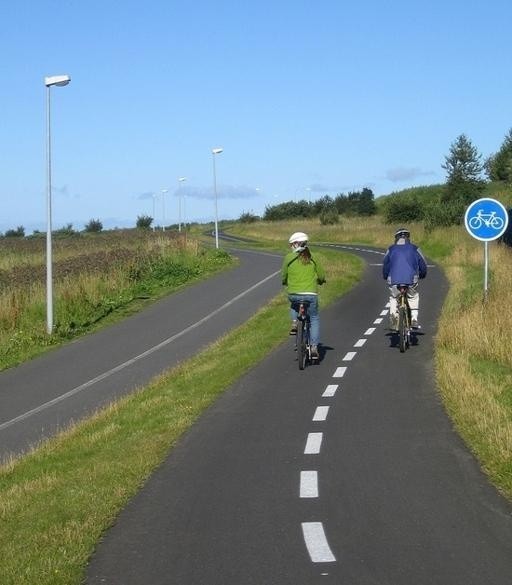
[390,283,421,353]
[290,301,319,370]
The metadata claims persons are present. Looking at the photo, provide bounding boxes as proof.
[383,228,427,335]
[281,232,326,360]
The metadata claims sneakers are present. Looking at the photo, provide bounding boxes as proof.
[410,320,421,327]
[310,345,319,356]
[389,323,397,331]
[292,320,298,331]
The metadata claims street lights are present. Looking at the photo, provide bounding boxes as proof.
[179,178,185,232]
[45,75,71,336]
[153,190,168,233]
[212,148,223,249]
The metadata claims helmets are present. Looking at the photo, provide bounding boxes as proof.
[395,229,410,238]
[289,232,308,244]
